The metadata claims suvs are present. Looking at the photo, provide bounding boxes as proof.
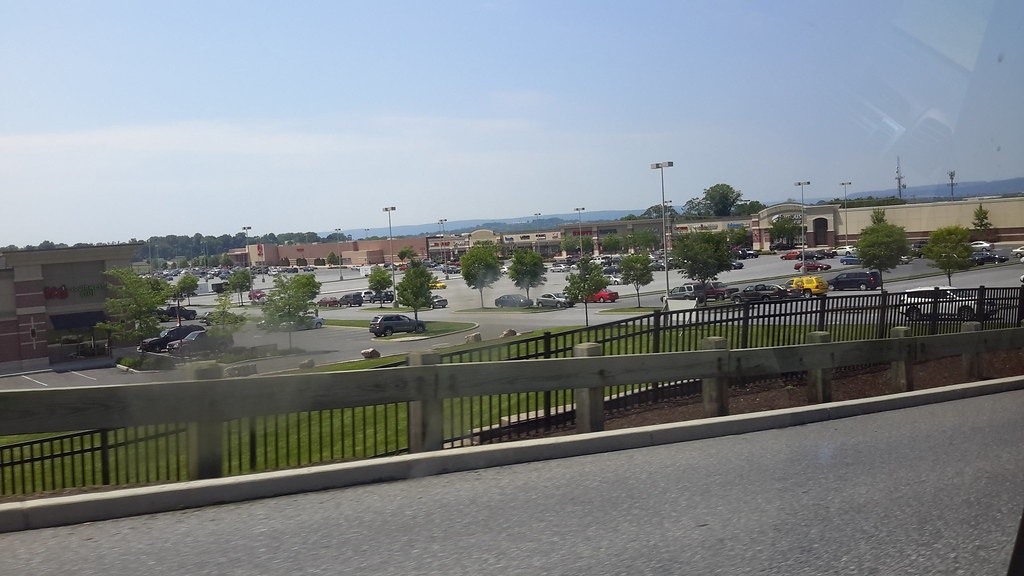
[137,325,234,358]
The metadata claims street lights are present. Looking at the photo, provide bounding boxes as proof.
[383,207,396,301]
[574,207,585,257]
[438,219,448,276]
[794,181,810,268]
[335,229,342,276]
[242,227,253,301]
[651,162,674,297]
[535,213,541,253]
[365,229,369,237]
[839,182,851,246]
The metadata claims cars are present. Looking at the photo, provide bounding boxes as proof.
[138,265,361,300]
[199,311,245,326]
[495,256,580,308]
[384,261,461,289]
[257,290,448,337]
[898,240,1024,322]
[660,242,928,302]
[587,249,680,303]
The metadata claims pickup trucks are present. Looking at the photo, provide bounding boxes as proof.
[158,306,197,322]
